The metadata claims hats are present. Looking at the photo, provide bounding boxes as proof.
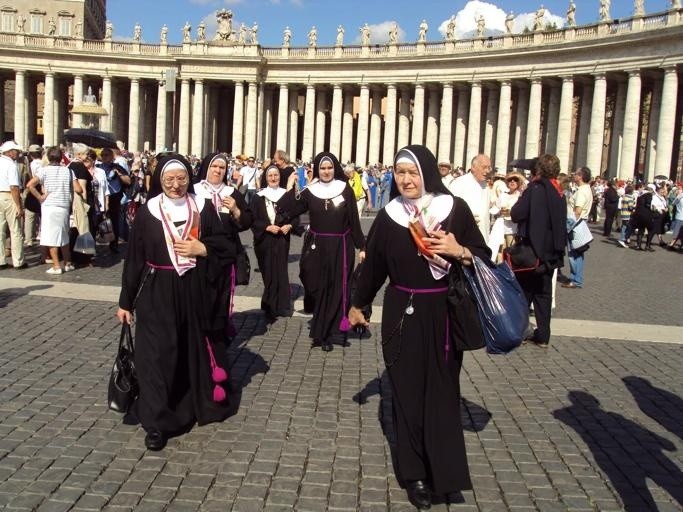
[26,144,43,156]
[0,140,23,153]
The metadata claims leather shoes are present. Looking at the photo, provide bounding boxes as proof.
[264,311,275,324]
[406,479,432,510]
[561,279,579,289]
[144,429,168,452]
[322,343,334,352]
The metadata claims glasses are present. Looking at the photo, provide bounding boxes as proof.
[507,176,520,182]
[162,175,188,188]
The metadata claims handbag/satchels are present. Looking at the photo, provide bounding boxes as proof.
[234,247,251,286]
[238,184,248,196]
[106,316,140,413]
[344,258,373,325]
[444,245,531,355]
[95,218,117,244]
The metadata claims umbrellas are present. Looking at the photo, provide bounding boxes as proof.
[62,127,117,154]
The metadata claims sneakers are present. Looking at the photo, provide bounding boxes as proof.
[4,248,11,257]
[64,265,76,272]
[45,266,63,275]
[0,262,14,270]
[616,240,630,248]
[522,334,549,349]
[635,246,656,252]
[14,262,29,271]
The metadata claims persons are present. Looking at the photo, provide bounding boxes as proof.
[117,151,236,451]
[438,154,526,265]
[557,166,682,250]
[225,153,275,205]
[192,153,254,339]
[185,154,202,175]
[287,160,313,190]
[510,152,560,348]
[274,150,294,189]
[286,151,367,351]
[341,162,393,219]
[15,1,683,49]
[564,167,592,288]
[348,145,491,508]
[0,140,158,276]
